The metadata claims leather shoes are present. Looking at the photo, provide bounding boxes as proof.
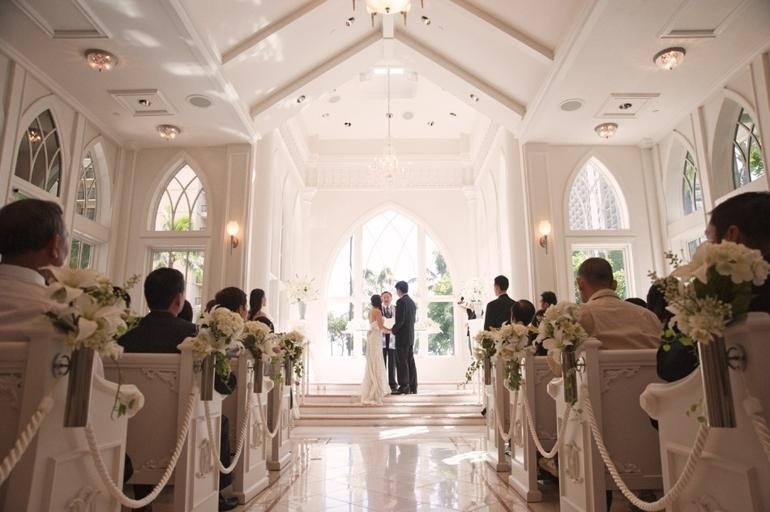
[391,388,418,394]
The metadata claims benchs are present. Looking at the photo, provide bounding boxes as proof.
[264,353,297,471]
[99,338,227,511]
[638,307,768,512]
[481,353,521,473]
[219,355,276,506]
[545,344,659,512]
[507,349,554,504]
[0,334,145,512]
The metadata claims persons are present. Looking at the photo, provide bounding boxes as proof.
[112,268,276,511]
[390,280,418,394]
[2,198,133,484]
[459,300,483,357]
[380,291,397,392]
[362,294,391,404]
[648,191,769,430]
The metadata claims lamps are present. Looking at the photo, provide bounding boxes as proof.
[158,124,181,141]
[654,47,687,72]
[25,125,50,145]
[538,220,555,252]
[225,221,242,249]
[343,0,424,30]
[596,122,621,138]
[84,48,119,73]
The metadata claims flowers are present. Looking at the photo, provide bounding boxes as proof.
[42,269,144,427]
[526,302,589,380]
[284,272,321,306]
[190,303,247,387]
[279,329,310,384]
[459,326,500,388]
[484,317,530,390]
[241,321,282,382]
[643,240,770,357]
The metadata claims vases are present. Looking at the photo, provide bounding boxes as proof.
[297,300,307,319]
